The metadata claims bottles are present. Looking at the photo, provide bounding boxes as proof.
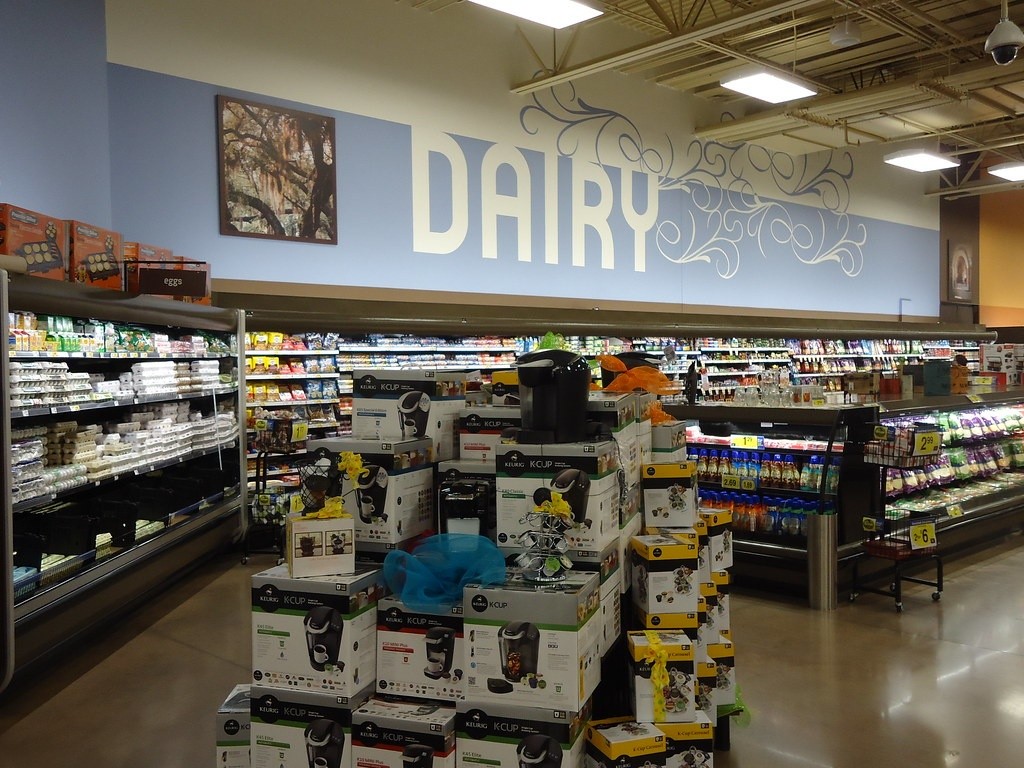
[688,448,841,494]
[696,490,833,539]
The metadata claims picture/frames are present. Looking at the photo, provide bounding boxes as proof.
[217,95,337,244]
[948,238,973,303]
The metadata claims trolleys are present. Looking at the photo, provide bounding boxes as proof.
[848,508,944,614]
[235,448,298,568]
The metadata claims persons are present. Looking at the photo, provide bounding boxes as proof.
[871,361,884,379]
[951,355,968,366]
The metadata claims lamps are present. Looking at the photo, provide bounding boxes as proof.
[466,0,605,30]
[987,161,1024,182]
[882,148,962,173]
[718,65,818,104]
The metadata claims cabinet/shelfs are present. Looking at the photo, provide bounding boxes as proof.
[0,270,1024,690]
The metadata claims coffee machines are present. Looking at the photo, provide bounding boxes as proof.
[513,347,591,445]
[356,465,389,521]
[497,621,541,682]
[304,719,345,768]
[423,627,455,680]
[435,476,492,556]
[548,468,591,528]
[516,734,564,768]
[396,390,431,437]
[302,607,344,672]
[401,744,434,768]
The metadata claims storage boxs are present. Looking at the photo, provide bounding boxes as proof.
[216,367,738,768]
[844,344,1024,404]
[0,203,71,282]
[62,219,126,291]
[173,256,213,308]
[122,241,174,300]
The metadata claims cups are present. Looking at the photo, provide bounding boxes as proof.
[314,757,328,768]
[360,496,375,515]
[756,369,829,408]
[514,510,577,577]
[734,386,758,407]
[404,419,417,436]
[313,645,328,663]
[426,657,443,673]
